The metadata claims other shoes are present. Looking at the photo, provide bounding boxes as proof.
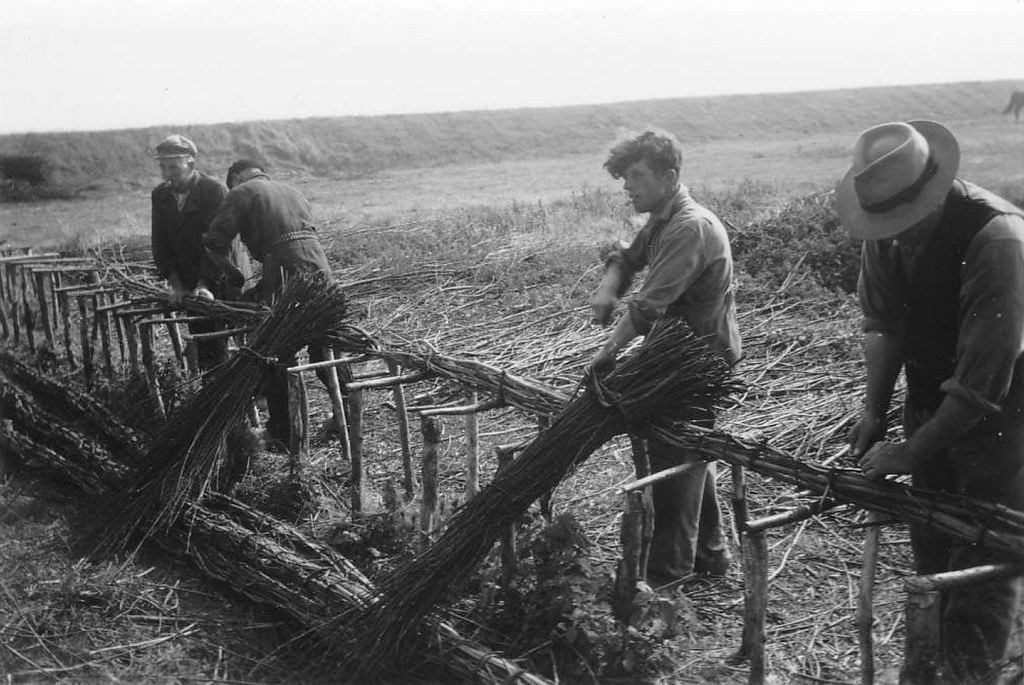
[617,556,693,583]
[695,544,733,573]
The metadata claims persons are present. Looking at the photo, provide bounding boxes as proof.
[585,128,744,604]
[199,157,355,444]
[149,134,257,388]
[833,118,1024,685]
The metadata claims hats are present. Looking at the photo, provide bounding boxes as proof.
[835,119,961,240]
[225,158,264,189]
[154,135,198,159]
